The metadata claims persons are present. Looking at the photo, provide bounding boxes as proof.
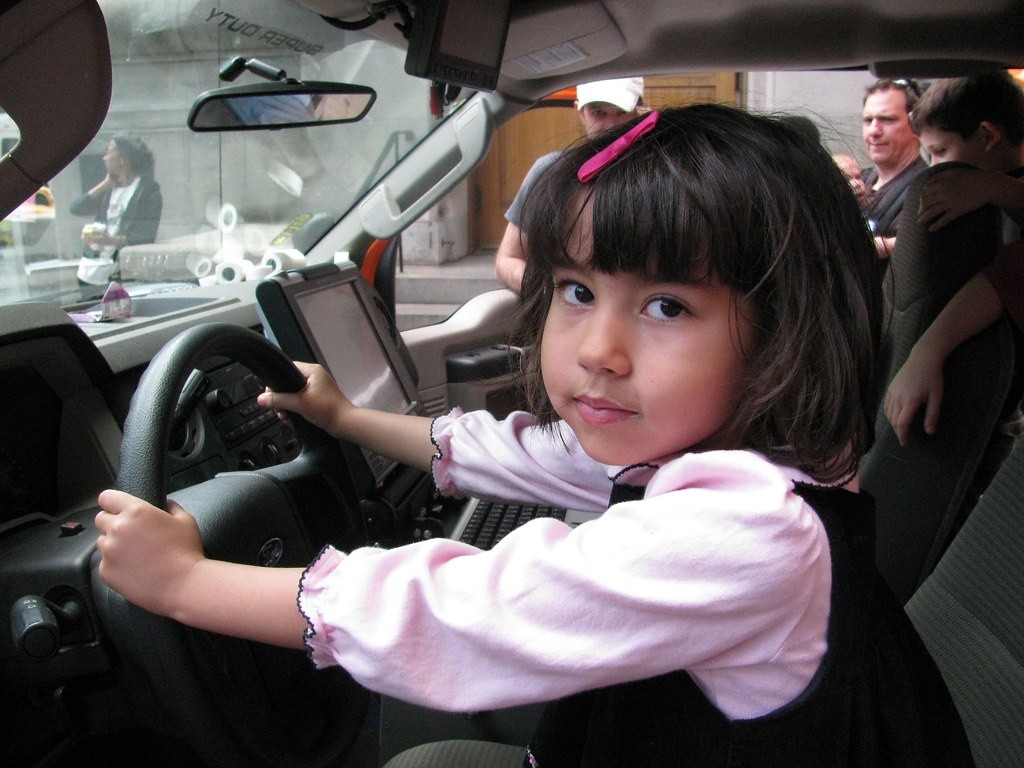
[95,103,920,768]
[495,76,655,305]
[70,135,161,300]
[837,70,1024,446]
[22,186,56,302]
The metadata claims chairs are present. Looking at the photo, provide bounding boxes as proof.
[855,160,1015,602]
[905,428,1024,768]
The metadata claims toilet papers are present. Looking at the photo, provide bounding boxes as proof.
[185,195,269,279]
[215,246,306,285]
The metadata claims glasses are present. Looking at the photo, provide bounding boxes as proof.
[893,78,920,100]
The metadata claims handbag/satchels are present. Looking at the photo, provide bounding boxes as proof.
[78,255,116,286]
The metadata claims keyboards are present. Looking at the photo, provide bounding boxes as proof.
[451,496,604,551]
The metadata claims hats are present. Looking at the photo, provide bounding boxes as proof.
[575,76,645,114]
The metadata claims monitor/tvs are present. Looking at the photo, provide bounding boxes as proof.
[260,262,434,518]
